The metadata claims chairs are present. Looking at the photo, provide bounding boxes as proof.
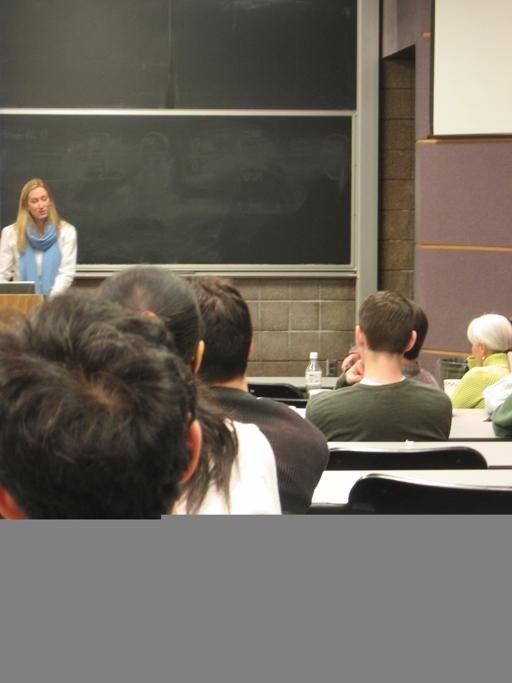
[0,372,512,514]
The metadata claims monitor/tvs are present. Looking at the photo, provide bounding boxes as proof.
[0,281,36,294]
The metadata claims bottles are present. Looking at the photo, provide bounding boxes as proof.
[304,350,323,398]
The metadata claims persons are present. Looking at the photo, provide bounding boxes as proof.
[1,263,328,521]
[305,291,511,440]
[1,178,77,303]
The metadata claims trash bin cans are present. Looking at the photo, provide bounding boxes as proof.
[440,358,469,391]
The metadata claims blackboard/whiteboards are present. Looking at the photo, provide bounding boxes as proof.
[3,108,356,277]
[2,1,357,111]
